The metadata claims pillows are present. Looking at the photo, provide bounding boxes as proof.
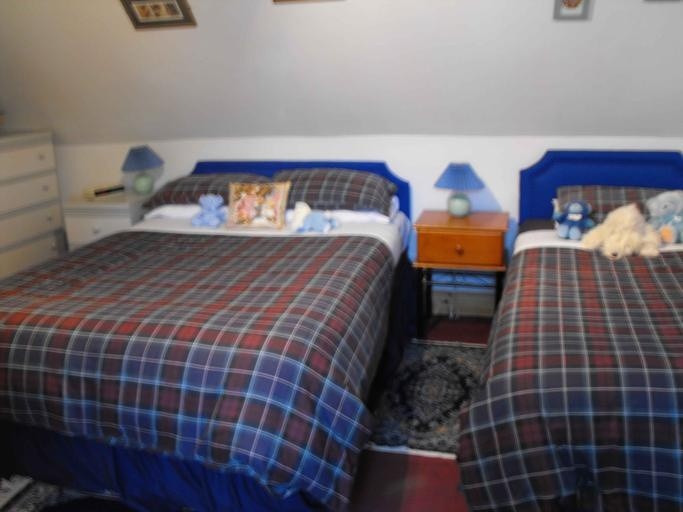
[548,181,669,234]
[143,168,398,223]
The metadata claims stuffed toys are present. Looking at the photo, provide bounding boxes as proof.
[646,187,683,245]
[578,203,661,259]
[552,200,594,239]
[284,202,337,235]
[192,192,223,228]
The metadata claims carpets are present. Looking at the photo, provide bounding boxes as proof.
[369,337,487,461]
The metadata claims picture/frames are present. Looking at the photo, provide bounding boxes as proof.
[121,1,199,32]
[553,1,591,22]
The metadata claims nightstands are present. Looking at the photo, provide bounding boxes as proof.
[412,209,508,336]
[60,187,160,252]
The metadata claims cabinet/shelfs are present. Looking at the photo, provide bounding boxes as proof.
[0,128,64,280]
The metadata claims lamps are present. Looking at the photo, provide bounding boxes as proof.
[432,162,485,217]
[120,145,166,194]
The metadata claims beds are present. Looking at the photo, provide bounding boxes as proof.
[0,159,412,510]
[460,143,681,512]
